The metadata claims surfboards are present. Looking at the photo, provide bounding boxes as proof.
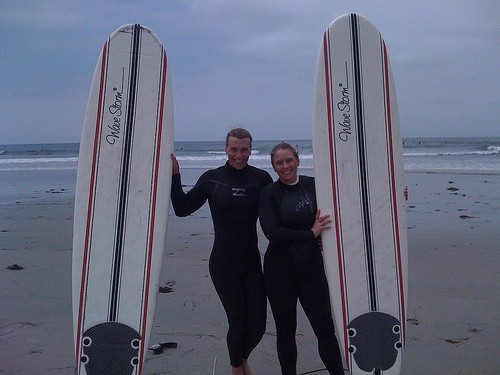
[311,13,410,375]
[70,24,174,375]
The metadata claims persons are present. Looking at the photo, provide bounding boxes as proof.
[258,143,408,375]
[170,128,323,375]
